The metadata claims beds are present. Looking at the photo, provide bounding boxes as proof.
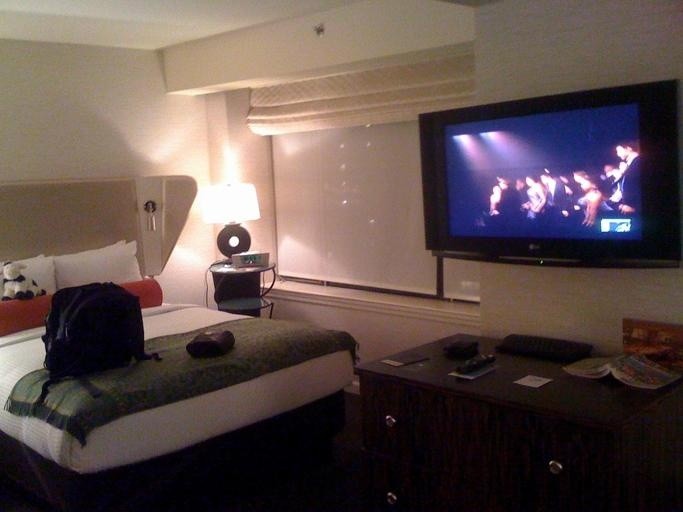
[0,176,358,512]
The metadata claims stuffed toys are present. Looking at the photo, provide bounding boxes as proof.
[0,261,46,301]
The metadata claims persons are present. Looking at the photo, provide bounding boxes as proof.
[490,141,641,240]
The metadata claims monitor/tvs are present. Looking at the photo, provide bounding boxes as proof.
[418,79,683,270]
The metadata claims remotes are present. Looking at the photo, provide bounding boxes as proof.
[453,352,496,374]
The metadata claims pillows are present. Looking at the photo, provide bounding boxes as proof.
[1,238,163,336]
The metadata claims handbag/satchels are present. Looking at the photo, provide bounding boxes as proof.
[186,330,235,358]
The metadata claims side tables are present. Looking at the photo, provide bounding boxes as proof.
[210,260,277,321]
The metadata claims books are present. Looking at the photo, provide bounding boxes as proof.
[561,354,682,389]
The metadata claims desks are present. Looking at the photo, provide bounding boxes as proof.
[353,329,682,509]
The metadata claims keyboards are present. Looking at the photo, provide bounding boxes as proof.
[495,333,596,362]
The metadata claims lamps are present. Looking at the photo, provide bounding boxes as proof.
[200,183,261,262]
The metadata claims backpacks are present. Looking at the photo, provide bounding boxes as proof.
[41,282,145,380]
